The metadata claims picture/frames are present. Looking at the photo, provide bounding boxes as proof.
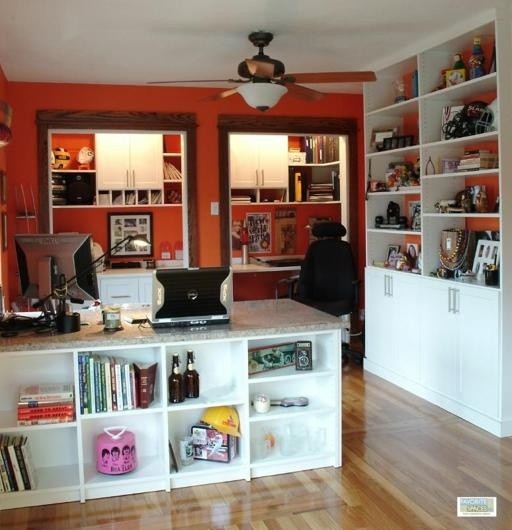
[107,212,155,257]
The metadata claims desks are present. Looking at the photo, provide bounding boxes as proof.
[230,262,301,301]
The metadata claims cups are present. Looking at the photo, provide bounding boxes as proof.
[179,436,196,466]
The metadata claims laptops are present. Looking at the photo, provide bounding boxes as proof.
[146,266,234,325]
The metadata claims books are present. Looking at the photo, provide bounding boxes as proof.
[1,431,39,495]
[288,135,341,203]
[378,222,406,230]
[410,201,422,229]
[231,200,251,204]
[453,142,498,173]
[77,350,160,416]
[15,383,75,428]
[231,195,251,201]
[99,161,183,206]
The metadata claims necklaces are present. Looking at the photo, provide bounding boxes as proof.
[437,225,477,275]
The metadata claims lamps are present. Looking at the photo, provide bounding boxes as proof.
[235,83,289,115]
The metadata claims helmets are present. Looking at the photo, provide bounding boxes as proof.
[442,101,494,140]
[203,406,241,438]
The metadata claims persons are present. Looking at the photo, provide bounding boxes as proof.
[407,245,419,269]
[230,219,243,251]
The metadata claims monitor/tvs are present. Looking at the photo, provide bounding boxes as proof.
[14,233,100,333]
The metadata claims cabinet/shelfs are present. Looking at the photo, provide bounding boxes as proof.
[230,133,340,207]
[1,298,352,510]
[360,5,511,439]
[94,267,152,304]
[50,130,183,207]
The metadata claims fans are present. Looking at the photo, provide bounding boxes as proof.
[143,32,377,114]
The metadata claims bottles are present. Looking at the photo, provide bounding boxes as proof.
[169,352,185,403]
[183,350,199,398]
